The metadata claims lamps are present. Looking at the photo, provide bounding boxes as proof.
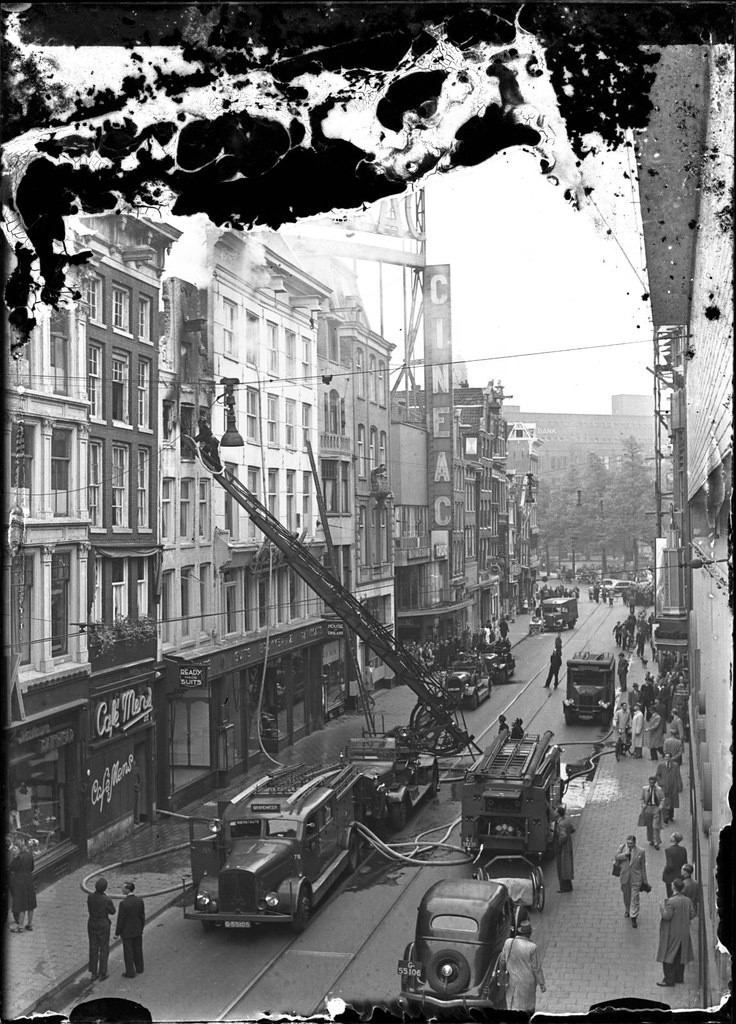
[525,474,535,503]
[219,375,245,449]
[575,489,583,506]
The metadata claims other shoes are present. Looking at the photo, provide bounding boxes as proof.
[626,911,629,917]
[650,841,654,845]
[557,889,572,893]
[656,978,683,986]
[91,973,109,982]
[664,818,673,824]
[648,758,658,761]
[632,917,637,928]
[122,973,135,978]
[633,754,642,759]
[655,844,659,850]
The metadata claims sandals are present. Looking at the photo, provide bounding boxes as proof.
[25,925,34,930]
[11,926,23,933]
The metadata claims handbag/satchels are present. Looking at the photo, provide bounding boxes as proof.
[637,810,647,826]
[498,969,509,989]
[642,882,651,892]
[611,859,621,876]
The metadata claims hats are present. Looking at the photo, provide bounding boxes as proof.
[516,717,524,726]
[617,652,625,657]
[631,683,639,687]
[648,705,657,711]
[518,920,536,933]
[635,702,641,707]
[645,677,651,681]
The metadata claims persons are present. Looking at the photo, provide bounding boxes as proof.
[656,752,683,824]
[681,863,699,922]
[4,834,21,925]
[656,878,695,987]
[401,581,688,741]
[631,703,643,759]
[662,832,687,898]
[644,705,664,761]
[10,839,38,933]
[14,782,33,826]
[87,878,116,982]
[614,835,647,928]
[553,807,576,893]
[114,882,146,978]
[614,702,630,756]
[501,921,546,1016]
[670,708,685,753]
[637,776,666,850]
[663,730,684,765]
[362,661,375,704]
[498,715,510,735]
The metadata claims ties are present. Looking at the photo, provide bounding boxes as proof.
[667,763,669,768]
[650,788,652,805]
[629,848,630,864]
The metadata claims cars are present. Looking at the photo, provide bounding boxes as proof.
[397,876,519,1009]
[601,578,636,596]
[441,644,515,709]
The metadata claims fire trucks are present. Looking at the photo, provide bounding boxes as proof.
[562,651,616,731]
[460,741,564,857]
[183,764,358,934]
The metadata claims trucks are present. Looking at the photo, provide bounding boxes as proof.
[540,596,578,631]
